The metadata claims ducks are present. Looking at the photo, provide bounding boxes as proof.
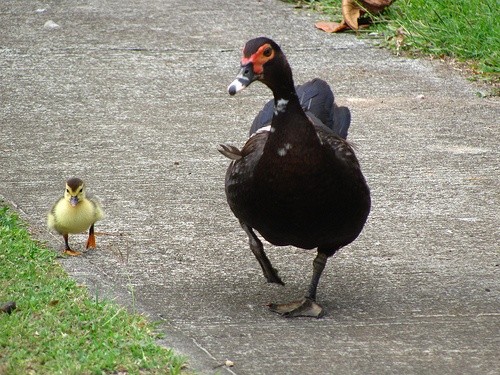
[224,37,371,318]
[44,179,105,257]
